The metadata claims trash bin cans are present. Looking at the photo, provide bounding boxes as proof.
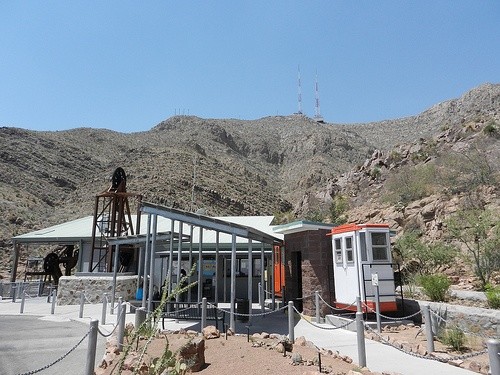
[235,297,249,323]
